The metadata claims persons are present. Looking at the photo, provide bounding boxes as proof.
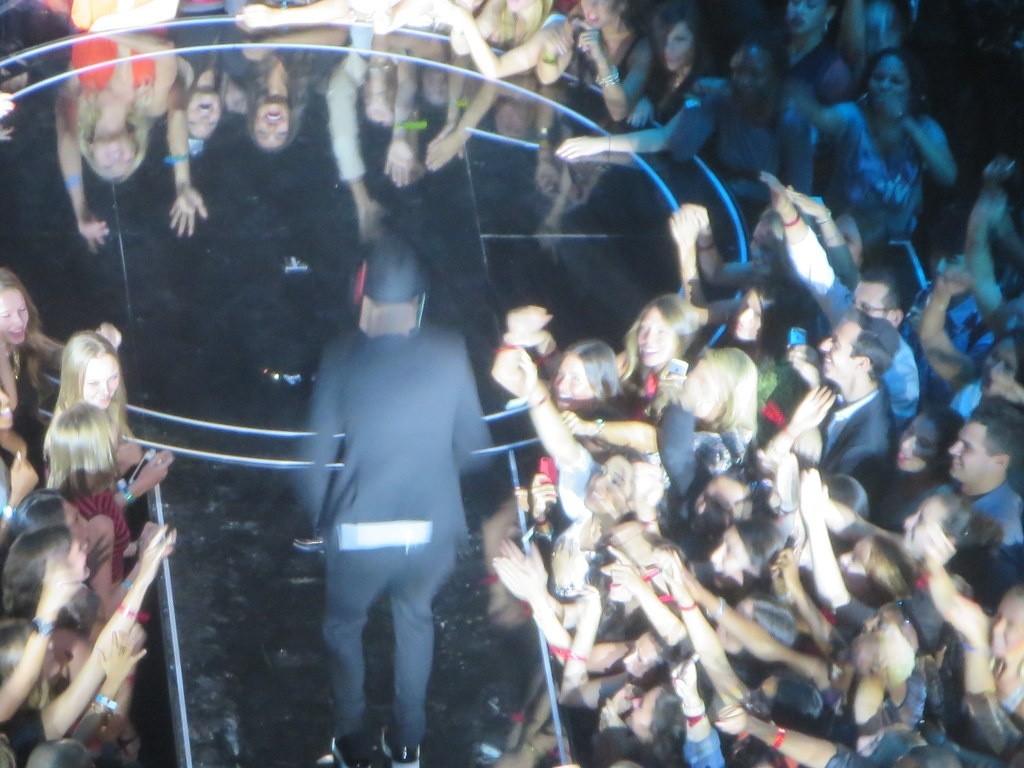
[0,0,1024,768]
[292,248,492,768]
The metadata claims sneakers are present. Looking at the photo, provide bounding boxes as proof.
[332,737,371,768]
[381,724,420,768]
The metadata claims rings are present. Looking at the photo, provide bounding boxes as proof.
[562,417,568,424]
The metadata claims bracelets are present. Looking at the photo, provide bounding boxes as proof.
[165,155,186,167]
[62,172,83,191]
[31,618,56,635]
[608,135,611,153]
[3,505,15,521]
[676,603,697,610]
[122,488,134,504]
[778,504,799,514]
[895,111,909,122]
[534,517,548,527]
[770,723,785,750]
[92,695,117,715]
[780,212,800,225]
[682,699,705,717]
[116,605,136,618]
[539,128,553,149]
[595,418,604,439]
[815,212,832,224]
[538,50,559,65]
[707,597,727,622]
[393,111,428,129]
[592,65,619,88]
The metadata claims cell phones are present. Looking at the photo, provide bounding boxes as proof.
[129,452,163,485]
[679,651,700,681]
[540,457,558,487]
[791,327,807,347]
[668,358,689,383]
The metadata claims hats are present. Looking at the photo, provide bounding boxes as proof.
[364,241,423,302]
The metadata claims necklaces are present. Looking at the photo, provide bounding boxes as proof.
[673,79,679,89]
[4,347,20,380]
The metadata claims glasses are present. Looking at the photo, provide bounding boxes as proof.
[844,293,887,315]
